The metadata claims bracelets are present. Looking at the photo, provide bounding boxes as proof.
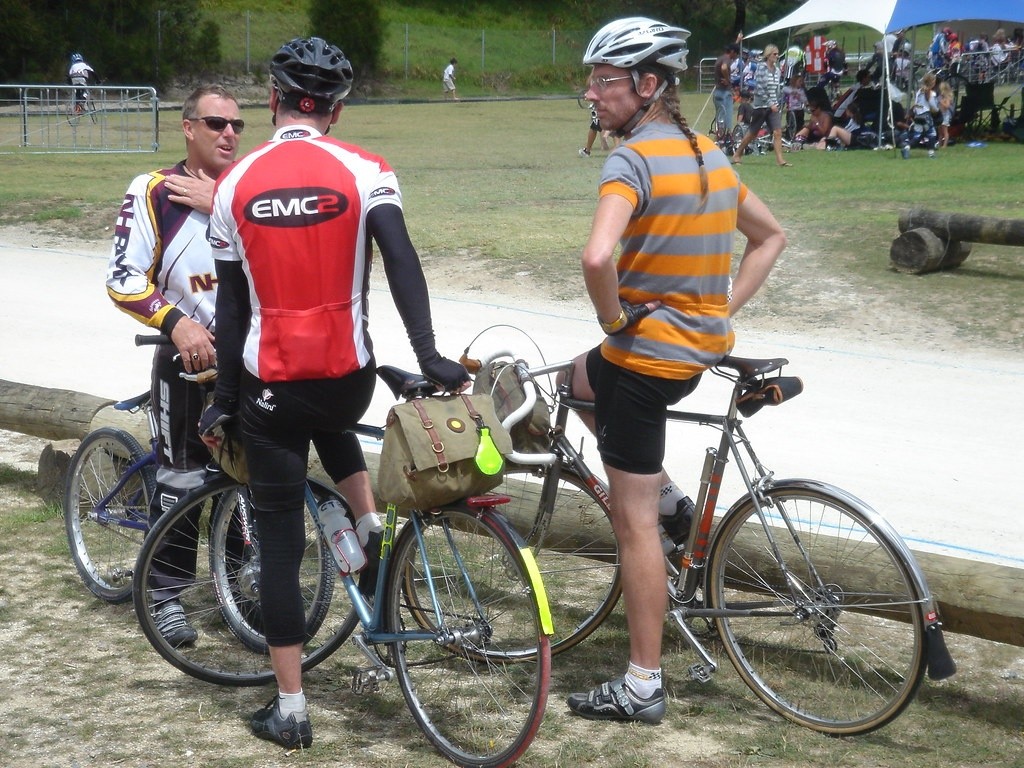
[600,310,626,331]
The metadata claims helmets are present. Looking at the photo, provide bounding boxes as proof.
[268,37,354,102]
[583,17,692,73]
[729,41,839,58]
[71,53,84,64]
[871,28,958,55]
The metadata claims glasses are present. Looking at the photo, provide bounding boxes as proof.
[772,53,778,55]
[589,73,640,91]
[187,116,244,134]
[811,108,816,113]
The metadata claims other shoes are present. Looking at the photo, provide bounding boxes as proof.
[901,149,909,159]
[579,148,590,157]
[78,103,85,111]
[929,153,937,158]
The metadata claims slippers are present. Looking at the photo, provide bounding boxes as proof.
[731,160,742,166]
[776,162,793,167]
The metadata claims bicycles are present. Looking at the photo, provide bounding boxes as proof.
[707,117,744,145]
[65,97,99,126]
[131,348,554,768]
[400,323,956,738]
[63,347,338,657]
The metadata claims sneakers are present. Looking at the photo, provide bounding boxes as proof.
[152,599,198,648]
[659,496,696,546]
[231,583,268,630]
[250,693,312,749]
[357,514,386,595]
[566,677,665,725]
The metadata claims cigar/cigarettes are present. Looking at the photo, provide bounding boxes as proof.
[285,749,296,757]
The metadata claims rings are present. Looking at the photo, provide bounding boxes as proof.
[184,189,187,195]
[192,354,198,360]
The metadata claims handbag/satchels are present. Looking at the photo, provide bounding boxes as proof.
[377,394,514,509]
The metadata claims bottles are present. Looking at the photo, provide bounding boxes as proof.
[656,516,676,555]
[317,494,365,573]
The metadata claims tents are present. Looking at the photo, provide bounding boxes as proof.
[742,0,1024,148]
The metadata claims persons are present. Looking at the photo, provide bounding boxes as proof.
[578,117,621,157]
[443,58,457,99]
[556,17,788,724]
[713,27,1024,167]
[68,53,101,111]
[106,84,254,646]
[198,37,471,750]
